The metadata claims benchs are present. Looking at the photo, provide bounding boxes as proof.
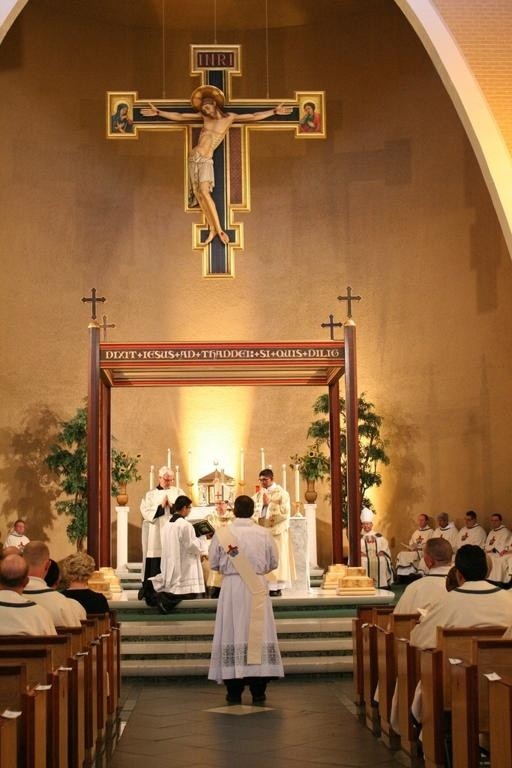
[0,611,121,768]
[351,605,512,768]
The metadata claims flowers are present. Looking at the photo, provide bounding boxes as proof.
[290,440,330,479]
[112,446,143,496]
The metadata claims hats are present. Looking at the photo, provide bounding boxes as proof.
[360,508,374,524]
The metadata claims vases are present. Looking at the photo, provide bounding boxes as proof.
[117,482,128,506]
[305,478,317,503]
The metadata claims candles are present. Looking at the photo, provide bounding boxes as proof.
[268,465,272,469]
[149,465,154,490]
[261,447,265,469]
[281,464,286,490]
[175,466,179,486]
[168,449,170,469]
[240,447,244,480]
[294,464,300,501]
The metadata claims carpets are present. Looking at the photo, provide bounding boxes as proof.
[110,673,403,767]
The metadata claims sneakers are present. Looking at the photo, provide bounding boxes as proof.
[223,692,268,705]
[138,579,172,615]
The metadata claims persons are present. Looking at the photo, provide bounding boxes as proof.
[298,102,322,132]
[207,495,285,705]
[360,507,393,588]
[112,103,133,133]
[394,511,512,590]
[374,537,512,743]
[0,520,110,636]
[138,465,208,613]
[141,85,293,247]
[250,469,296,597]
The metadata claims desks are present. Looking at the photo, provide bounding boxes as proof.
[189,516,310,595]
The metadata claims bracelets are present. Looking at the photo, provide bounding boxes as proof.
[274,109,277,116]
[155,110,159,117]
